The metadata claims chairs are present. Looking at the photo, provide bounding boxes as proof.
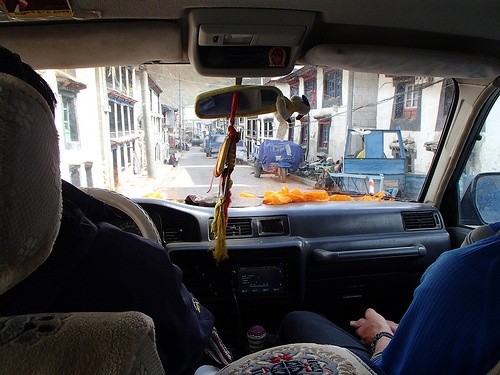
[0,72,165,375]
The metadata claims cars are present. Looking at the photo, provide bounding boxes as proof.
[234,140,248,163]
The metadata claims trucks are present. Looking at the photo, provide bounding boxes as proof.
[332,128,464,202]
[252,138,301,182]
[205,134,225,157]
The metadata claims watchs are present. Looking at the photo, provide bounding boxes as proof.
[371,331,392,353]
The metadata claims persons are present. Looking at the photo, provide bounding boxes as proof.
[279,220,498,375]
[0,46,233,375]
